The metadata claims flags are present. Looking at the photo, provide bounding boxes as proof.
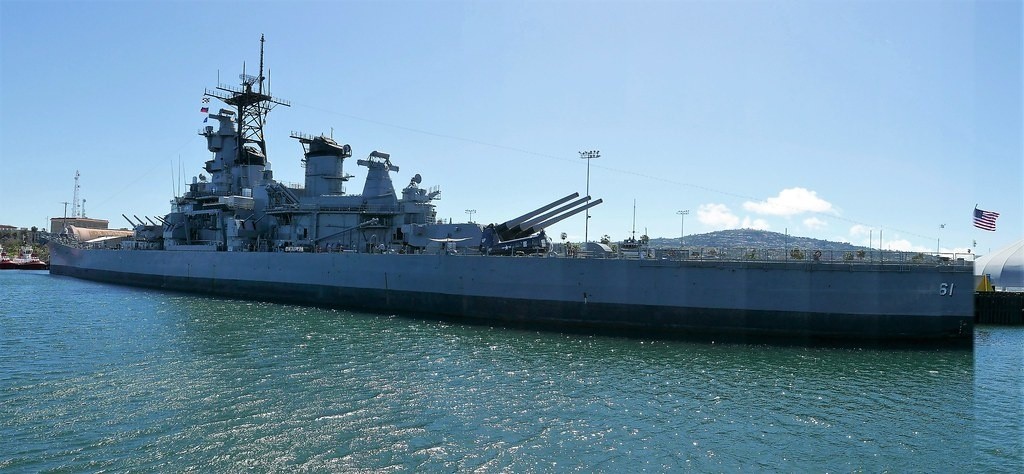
[202,97,210,103]
[972,206,1000,231]
[200,107,208,113]
[203,117,207,123]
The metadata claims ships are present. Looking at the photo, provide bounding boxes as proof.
[49,33,975,350]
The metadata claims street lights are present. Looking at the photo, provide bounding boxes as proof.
[579,150,601,242]
[676,210,689,246]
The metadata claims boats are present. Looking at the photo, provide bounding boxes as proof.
[0,244,47,270]
